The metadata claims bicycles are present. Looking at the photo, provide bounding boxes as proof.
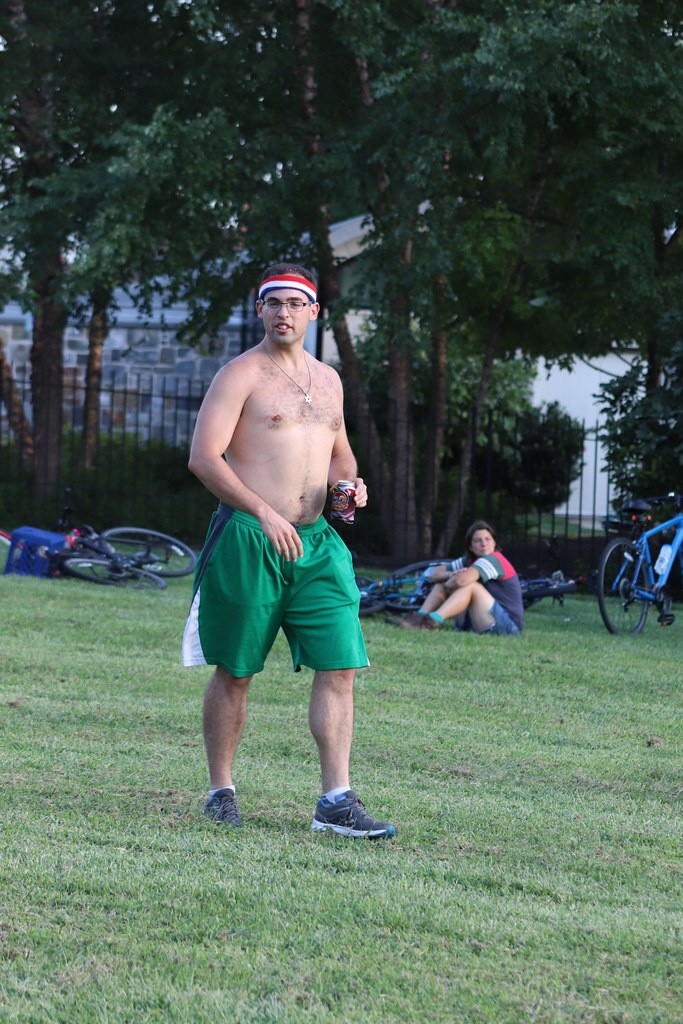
[4,524,198,590]
[351,558,598,621]
[598,493,683,636]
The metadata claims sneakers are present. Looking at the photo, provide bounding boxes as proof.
[202,788,241,824]
[384,611,420,626]
[401,614,439,629]
[311,789,398,838]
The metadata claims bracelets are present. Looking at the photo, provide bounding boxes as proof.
[446,572,450,578]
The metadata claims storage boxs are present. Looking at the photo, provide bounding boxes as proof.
[4,527,66,578]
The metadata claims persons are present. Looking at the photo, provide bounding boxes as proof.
[183,263,395,841]
[386,521,524,635]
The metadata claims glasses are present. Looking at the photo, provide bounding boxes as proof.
[260,299,312,312]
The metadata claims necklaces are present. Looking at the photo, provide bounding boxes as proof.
[262,345,312,404]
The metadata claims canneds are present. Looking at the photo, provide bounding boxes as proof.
[329,480,356,524]
